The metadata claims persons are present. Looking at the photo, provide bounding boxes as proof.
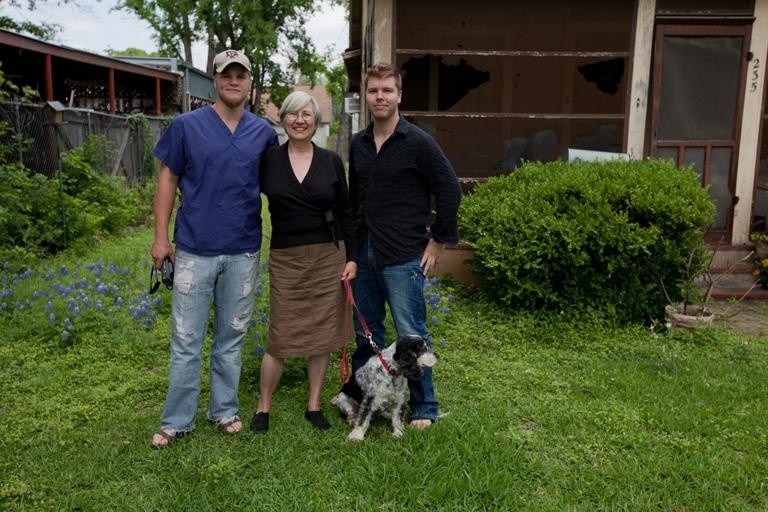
[250,90,359,435]
[330,62,462,427]
[149,48,278,445]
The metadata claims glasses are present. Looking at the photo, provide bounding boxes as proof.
[286,111,315,121]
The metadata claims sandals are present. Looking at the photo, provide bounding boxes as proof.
[210,415,243,436]
[150,429,190,449]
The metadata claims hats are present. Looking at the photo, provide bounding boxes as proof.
[212,50,253,76]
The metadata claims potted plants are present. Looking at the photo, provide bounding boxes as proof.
[659,235,764,331]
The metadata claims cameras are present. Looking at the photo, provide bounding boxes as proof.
[161,256,174,287]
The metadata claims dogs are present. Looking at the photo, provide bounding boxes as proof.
[335,334,441,443]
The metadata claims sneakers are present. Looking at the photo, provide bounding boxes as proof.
[251,411,269,435]
[305,407,331,434]
